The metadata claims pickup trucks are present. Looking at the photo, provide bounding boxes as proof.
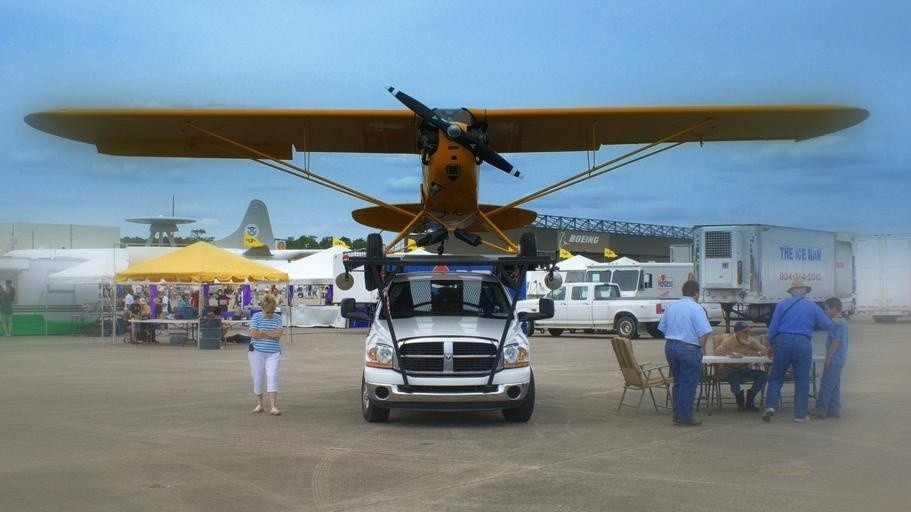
[513,281,682,337]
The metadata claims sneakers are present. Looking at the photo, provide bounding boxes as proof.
[674,390,840,426]
[270,408,281,415]
[253,405,264,412]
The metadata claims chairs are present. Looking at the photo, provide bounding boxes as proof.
[610,334,674,416]
[666,330,829,412]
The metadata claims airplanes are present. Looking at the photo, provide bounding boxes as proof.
[23,83,872,290]
[0,198,328,306]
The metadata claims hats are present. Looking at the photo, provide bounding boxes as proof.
[787,278,811,294]
[735,322,752,332]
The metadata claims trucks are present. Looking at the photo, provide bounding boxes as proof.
[526,260,755,333]
[693,224,910,327]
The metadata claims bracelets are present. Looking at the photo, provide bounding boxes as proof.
[758,351,762,357]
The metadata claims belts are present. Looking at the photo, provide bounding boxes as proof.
[666,339,682,343]
[776,332,811,339]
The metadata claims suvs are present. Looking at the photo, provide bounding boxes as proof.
[341,265,555,424]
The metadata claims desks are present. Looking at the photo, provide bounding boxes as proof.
[127,318,201,350]
[220,319,251,348]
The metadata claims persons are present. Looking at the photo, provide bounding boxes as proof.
[806,295,848,420]
[246,292,285,419]
[760,277,842,424]
[4,278,15,301]
[0,285,12,338]
[714,322,770,412]
[656,279,715,427]
[118,283,290,345]
